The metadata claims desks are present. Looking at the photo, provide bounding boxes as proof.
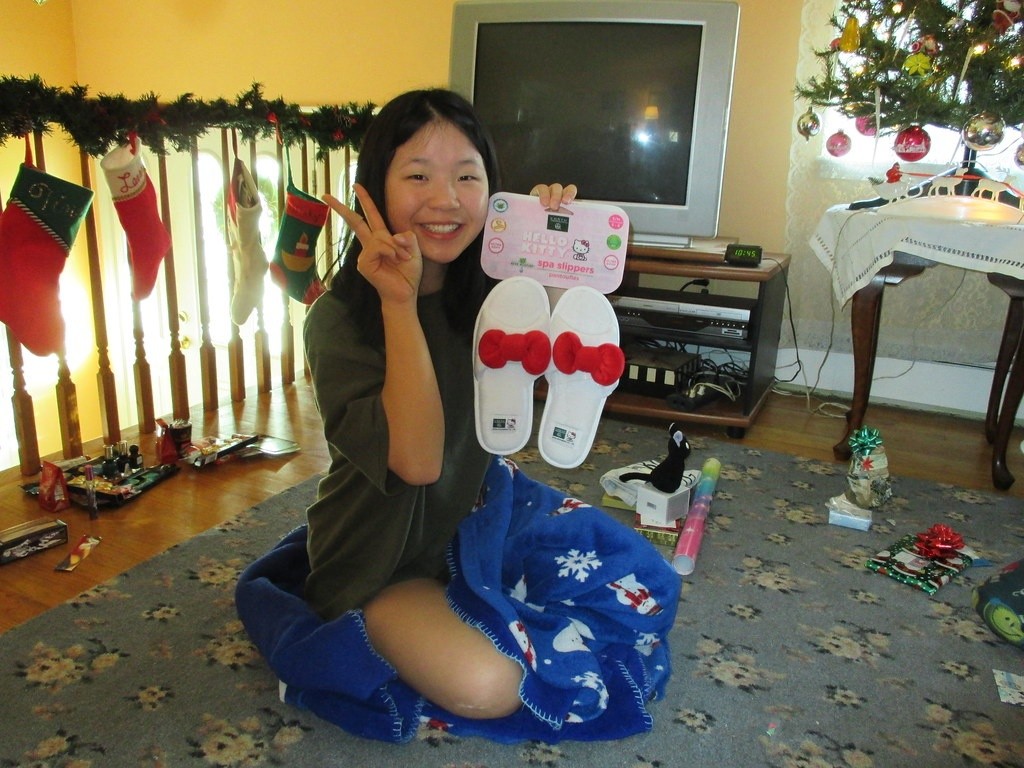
[810,198,1024,489]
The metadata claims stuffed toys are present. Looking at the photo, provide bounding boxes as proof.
[620,423,691,493]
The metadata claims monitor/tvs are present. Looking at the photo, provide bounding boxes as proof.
[446,0,742,247]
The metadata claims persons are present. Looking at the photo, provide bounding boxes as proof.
[304,87,579,722]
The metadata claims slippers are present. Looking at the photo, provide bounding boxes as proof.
[473,276,625,468]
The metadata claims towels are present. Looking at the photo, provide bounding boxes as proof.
[598,459,701,508]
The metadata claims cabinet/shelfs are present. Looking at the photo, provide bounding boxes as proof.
[534,238,794,437]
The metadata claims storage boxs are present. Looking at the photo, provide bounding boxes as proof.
[0,518,68,566]
[828,505,873,531]
[601,480,691,544]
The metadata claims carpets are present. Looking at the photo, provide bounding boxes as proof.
[0,404,1024,768]
